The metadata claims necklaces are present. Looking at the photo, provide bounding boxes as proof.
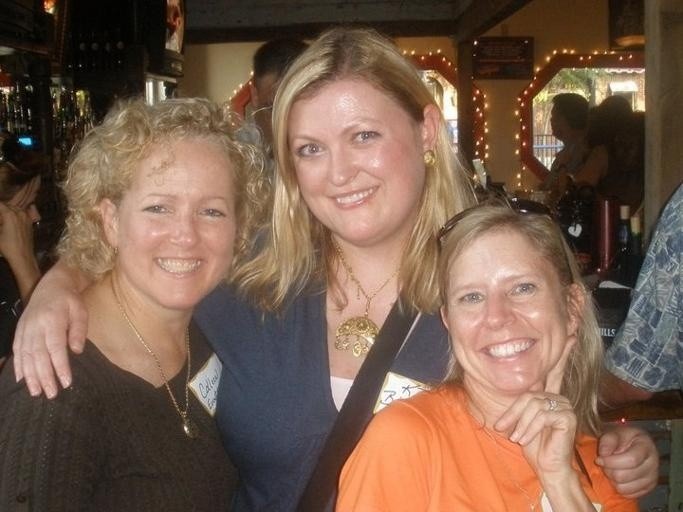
[106,274,201,438]
[327,237,400,357]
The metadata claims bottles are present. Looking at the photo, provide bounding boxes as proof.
[613,201,645,280]
[1,82,97,164]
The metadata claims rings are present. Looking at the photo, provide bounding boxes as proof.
[544,395,558,412]
[12,206,21,212]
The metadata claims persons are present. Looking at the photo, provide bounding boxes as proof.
[9,26,662,512]
[517,89,618,252]
[335,199,640,512]
[597,178,683,419]
[235,36,314,216]
[3,98,277,512]
[0,129,56,361]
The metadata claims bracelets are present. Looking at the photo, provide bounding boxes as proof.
[595,392,617,413]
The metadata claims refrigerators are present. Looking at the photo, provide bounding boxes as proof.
[63,2,187,112]
